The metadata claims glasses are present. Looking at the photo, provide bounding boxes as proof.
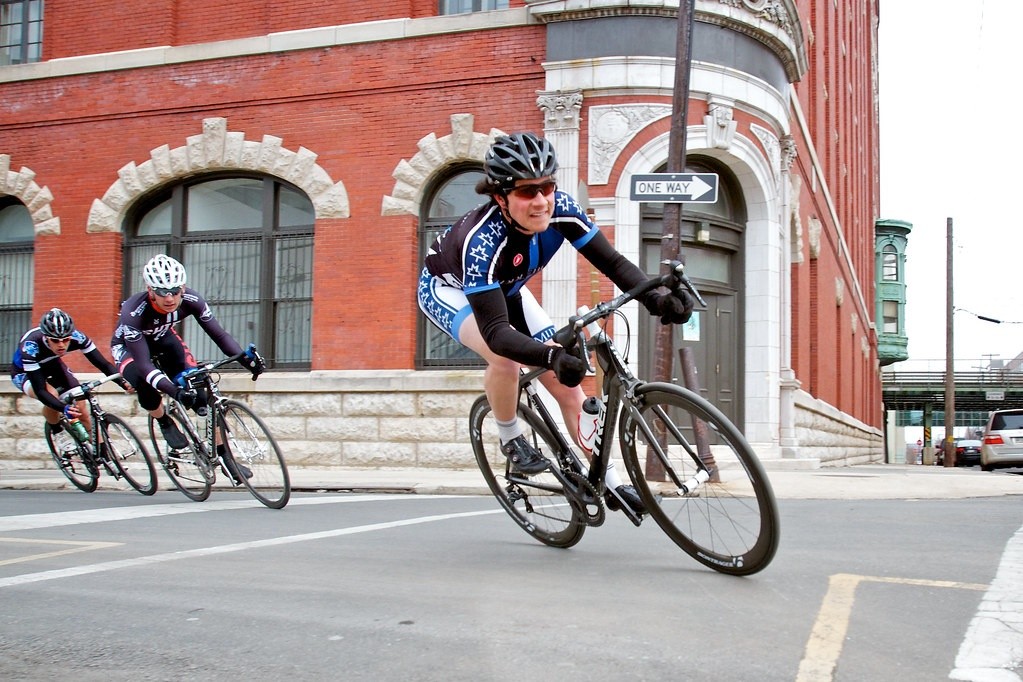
[148,286,181,298]
[501,179,556,199]
[47,336,71,344]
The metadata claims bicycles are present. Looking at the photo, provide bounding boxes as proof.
[144,342,294,510]
[469,259,783,578]
[44,374,159,497]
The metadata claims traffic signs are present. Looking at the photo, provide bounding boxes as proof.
[626,171,719,206]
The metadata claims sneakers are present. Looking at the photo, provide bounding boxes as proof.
[222,454,255,479]
[500,434,552,473]
[161,418,189,449]
[606,485,662,514]
[53,429,77,452]
[105,461,129,477]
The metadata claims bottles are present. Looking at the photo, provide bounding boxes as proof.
[70,418,90,442]
[577,397,601,452]
[197,406,208,437]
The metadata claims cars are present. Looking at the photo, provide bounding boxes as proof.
[952,439,983,466]
[976,408,1023,473]
[934,437,965,466]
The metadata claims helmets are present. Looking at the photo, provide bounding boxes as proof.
[143,253,187,289]
[40,308,74,338]
[484,131,559,185]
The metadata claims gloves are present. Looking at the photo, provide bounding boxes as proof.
[175,388,197,409]
[552,345,592,388]
[243,353,266,375]
[655,288,694,326]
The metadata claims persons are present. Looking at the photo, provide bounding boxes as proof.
[418,134,693,514]
[11,308,135,475]
[110,253,265,481]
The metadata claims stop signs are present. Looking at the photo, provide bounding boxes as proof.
[917,439,922,445]
